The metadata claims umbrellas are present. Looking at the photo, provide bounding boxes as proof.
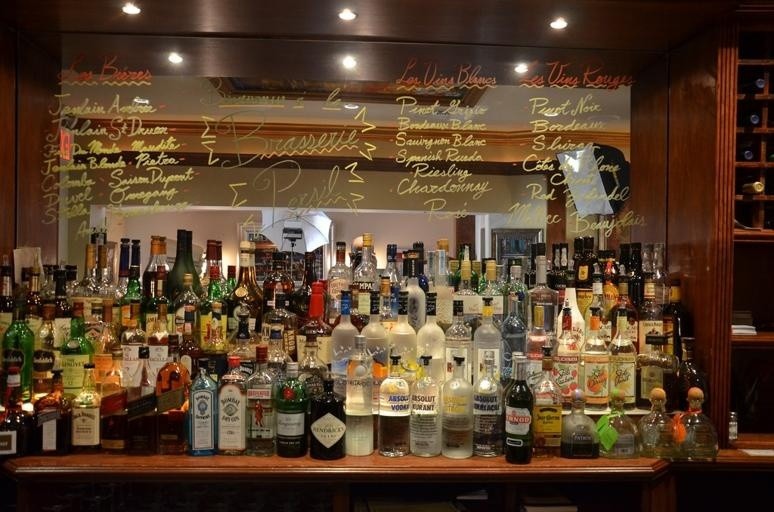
[260,207,334,255]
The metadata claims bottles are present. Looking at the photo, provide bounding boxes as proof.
[728,411,738,441]
[740,72,766,197]
[0,228,719,464]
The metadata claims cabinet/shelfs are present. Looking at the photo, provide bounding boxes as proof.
[0,438,701,511]
[670,28,773,467]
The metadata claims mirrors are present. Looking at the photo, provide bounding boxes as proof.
[16,33,670,304]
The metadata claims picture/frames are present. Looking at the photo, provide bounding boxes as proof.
[491,225,547,264]
[236,223,337,296]
